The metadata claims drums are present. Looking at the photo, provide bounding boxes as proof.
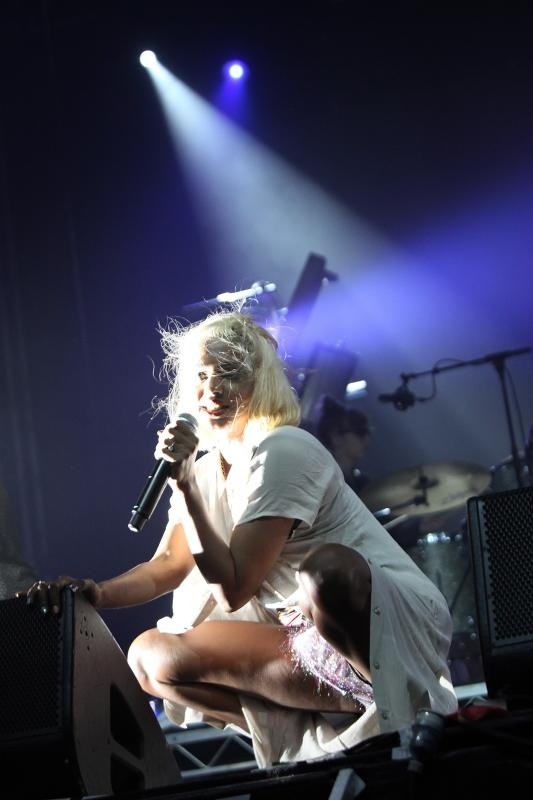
[395,525,486,647]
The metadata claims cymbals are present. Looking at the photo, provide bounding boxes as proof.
[489,451,533,491]
[356,461,501,518]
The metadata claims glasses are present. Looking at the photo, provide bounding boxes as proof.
[349,426,371,437]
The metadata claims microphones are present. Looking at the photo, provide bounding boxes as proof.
[125,411,199,535]
[376,388,424,413]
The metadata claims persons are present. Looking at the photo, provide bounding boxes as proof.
[15,289,457,765]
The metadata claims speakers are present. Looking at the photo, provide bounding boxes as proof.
[0,585,185,800]
[465,484,533,710]
